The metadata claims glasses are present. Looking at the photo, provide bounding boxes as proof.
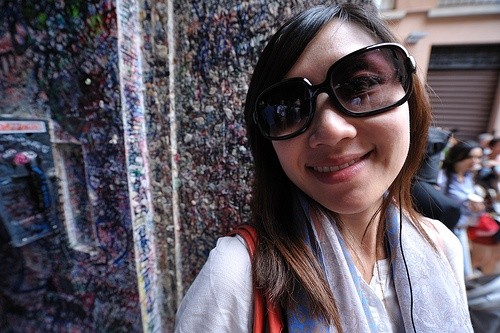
[253,41,416,140]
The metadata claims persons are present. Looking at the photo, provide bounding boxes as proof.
[413,126,500,333]
[172,3,474,333]
